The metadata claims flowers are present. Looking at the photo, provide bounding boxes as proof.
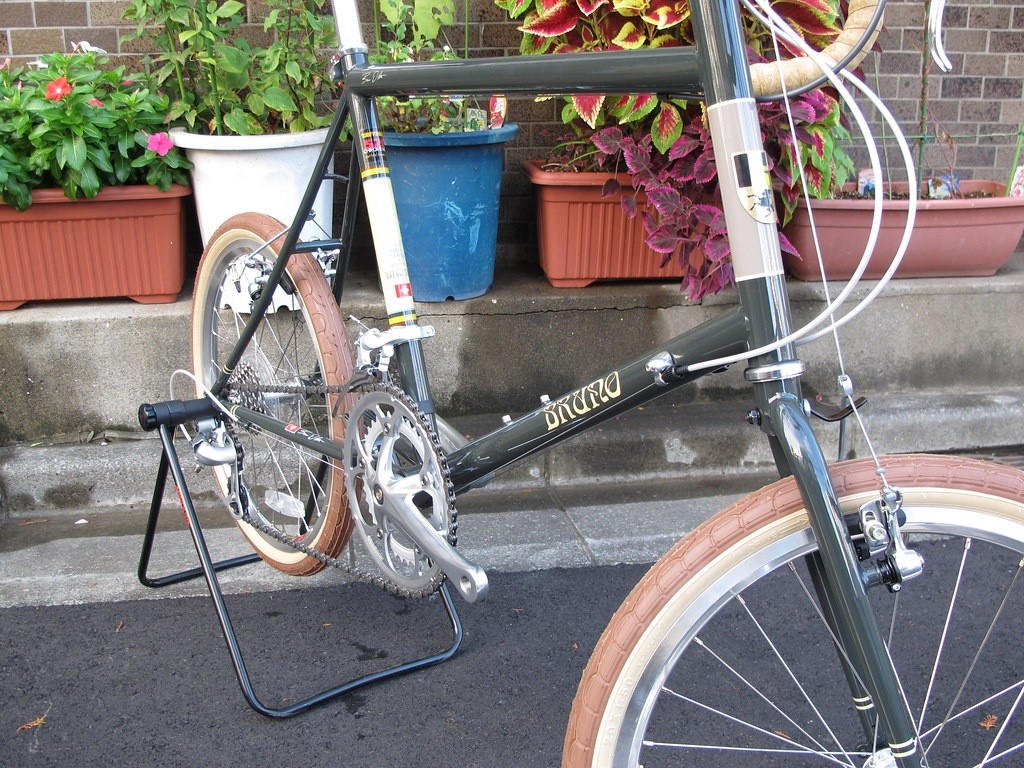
[0,38,195,209]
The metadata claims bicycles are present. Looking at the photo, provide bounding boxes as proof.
[133,2,1022,767]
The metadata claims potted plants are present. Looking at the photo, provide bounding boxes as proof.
[495,0,865,303]
[118,0,353,314]
[774,1,1024,277]
[341,0,519,303]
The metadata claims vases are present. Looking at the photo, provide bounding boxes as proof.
[0,182,191,314]
